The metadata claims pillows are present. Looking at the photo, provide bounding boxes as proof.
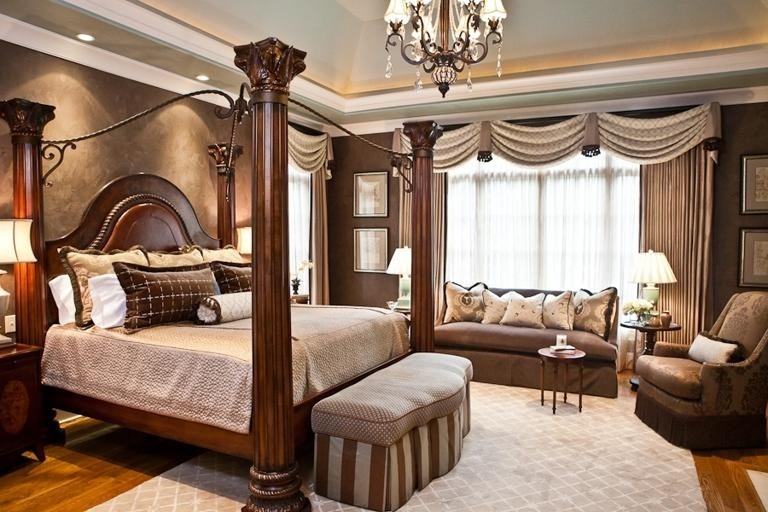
[572,286,618,343]
[195,291,252,326]
[144,244,205,269]
[441,280,489,323]
[542,289,575,332]
[209,260,253,293]
[688,331,743,365]
[499,292,547,330]
[47,273,76,326]
[87,273,128,331]
[111,260,219,336]
[191,243,244,264]
[480,289,516,325]
[56,244,151,332]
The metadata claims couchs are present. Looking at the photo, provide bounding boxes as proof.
[434,288,619,400]
[632,289,767,453]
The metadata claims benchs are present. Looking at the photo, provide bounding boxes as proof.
[308,350,476,512]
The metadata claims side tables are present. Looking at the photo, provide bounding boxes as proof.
[619,320,682,387]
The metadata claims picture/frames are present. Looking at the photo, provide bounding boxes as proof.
[353,227,388,275]
[352,171,388,218]
[739,151,768,216]
[736,225,768,289]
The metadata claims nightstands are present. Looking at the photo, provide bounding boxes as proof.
[0,340,48,464]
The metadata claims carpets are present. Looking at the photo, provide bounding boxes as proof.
[80,377,710,512]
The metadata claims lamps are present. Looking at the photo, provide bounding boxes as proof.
[624,248,678,326]
[0,218,38,346]
[379,0,509,101]
[384,244,412,308]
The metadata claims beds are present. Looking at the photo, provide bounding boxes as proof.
[0,34,444,512]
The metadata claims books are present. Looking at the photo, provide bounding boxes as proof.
[549,344,576,355]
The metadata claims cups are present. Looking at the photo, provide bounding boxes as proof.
[556,334,567,346]
